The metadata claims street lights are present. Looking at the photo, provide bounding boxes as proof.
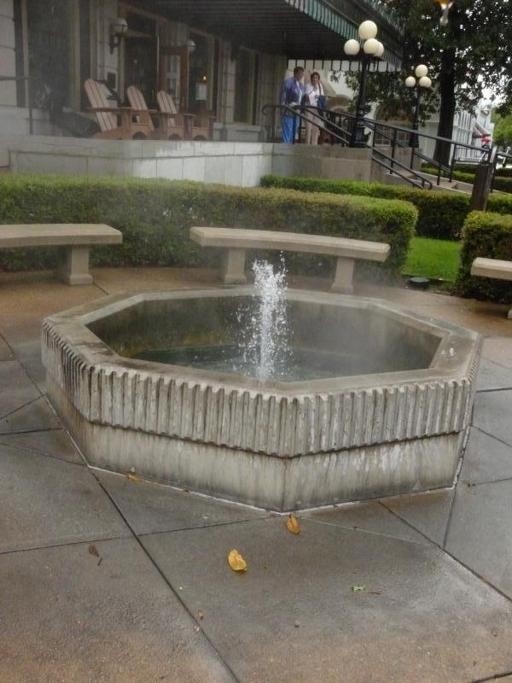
[340,15,386,147]
[406,61,434,149]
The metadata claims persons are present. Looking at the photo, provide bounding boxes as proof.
[280,66,306,143]
[481,139,490,161]
[304,70,324,146]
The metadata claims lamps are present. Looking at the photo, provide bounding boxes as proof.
[107,13,128,50]
[182,35,197,58]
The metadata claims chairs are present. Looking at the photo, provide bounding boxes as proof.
[82,77,214,140]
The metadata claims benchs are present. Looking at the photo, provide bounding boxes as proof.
[469,256,511,318]
[1,220,125,287]
[187,224,392,297]
